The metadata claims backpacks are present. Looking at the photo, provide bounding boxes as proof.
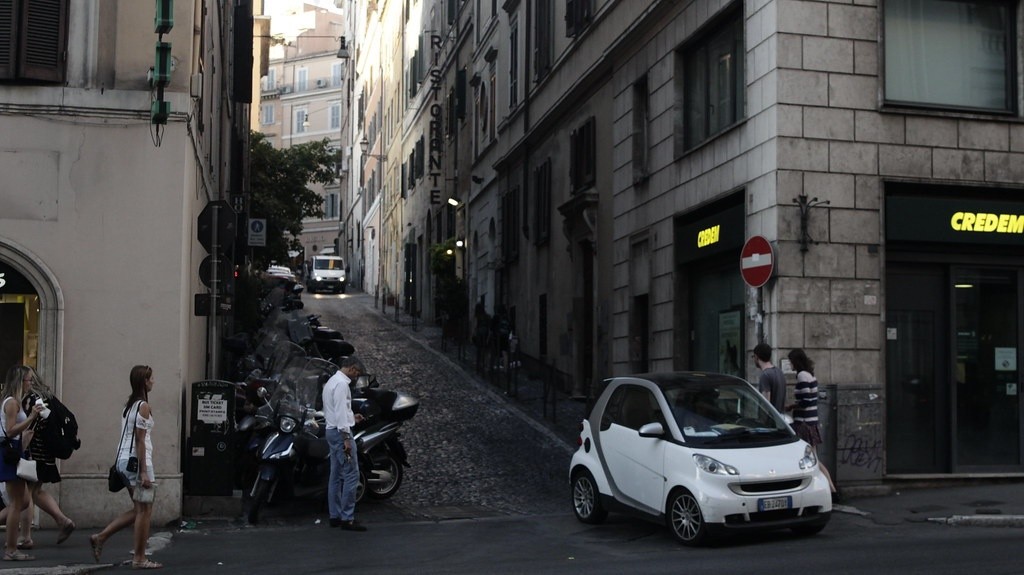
[498,313,510,337]
[28,392,77,460]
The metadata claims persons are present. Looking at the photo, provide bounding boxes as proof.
[469,302,507,387]
[89,366,165,570]
[320,356,368,532]
[0,364,76,562]
[784,348,822,454]
[753,342,786,429]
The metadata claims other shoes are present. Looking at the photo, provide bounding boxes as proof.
[341,521,366,531]
[832,490,841,503]
[330,518,345,527]
[5,539,33,548]
[56,519,75,543]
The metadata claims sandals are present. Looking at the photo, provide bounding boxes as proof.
[3,550,35,560]
[133,559,162,569]
[88,534,102,562]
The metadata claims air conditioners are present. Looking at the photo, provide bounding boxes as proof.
[317,79,328,88]
[279,85,292,96]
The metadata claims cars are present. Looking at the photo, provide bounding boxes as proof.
[569,370,833,545]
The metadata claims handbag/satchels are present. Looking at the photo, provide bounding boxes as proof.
[132,480,157,503]
[7,442,20,465]
[108,465,126,492]
[16,458,38,482]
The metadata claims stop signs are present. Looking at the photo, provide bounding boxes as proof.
[740,235,773,288]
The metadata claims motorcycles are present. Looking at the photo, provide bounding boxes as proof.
[222,273,420,524]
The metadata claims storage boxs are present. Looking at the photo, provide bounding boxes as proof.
[369,389,418,419]
[315,327,379,397]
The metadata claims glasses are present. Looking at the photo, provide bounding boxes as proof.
[23,378,33,382]
[145,365,151,374]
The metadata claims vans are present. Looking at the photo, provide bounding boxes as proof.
[307,255,345,294]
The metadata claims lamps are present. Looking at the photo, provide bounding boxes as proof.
[338,36,353,57]
[447,196,460,206]
[360,136,388,160]
